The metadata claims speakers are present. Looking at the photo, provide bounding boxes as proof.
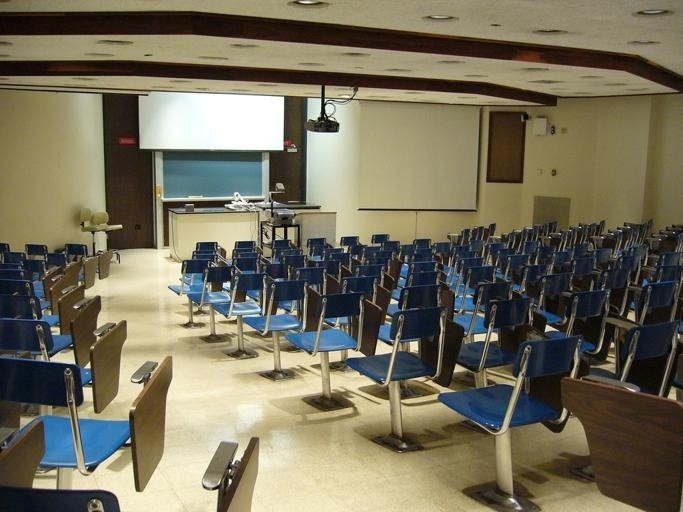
[532,118,548,136]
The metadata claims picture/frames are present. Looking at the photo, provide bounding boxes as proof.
[483,109,528,184]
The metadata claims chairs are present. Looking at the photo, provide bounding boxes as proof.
[168,218,682,510]
[89,210,124,263]
[0,242,260,512]
[76,206,105,264]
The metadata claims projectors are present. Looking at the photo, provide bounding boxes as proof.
[304,117,339,133]
